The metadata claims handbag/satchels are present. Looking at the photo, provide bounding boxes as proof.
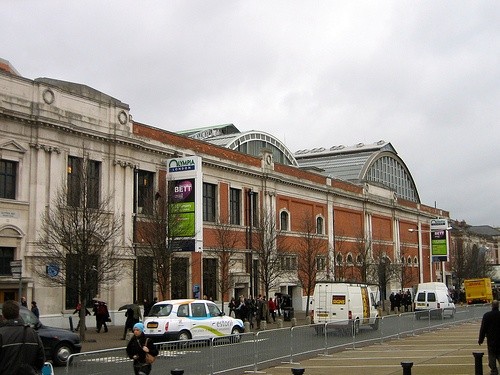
[146,353,155,364]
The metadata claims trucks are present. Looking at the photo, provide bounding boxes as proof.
[463,278,493,304]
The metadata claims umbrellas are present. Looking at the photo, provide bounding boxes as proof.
[274,292,292,298]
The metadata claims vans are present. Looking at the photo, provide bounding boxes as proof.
[311,283,380,336]
[414,282,456,321]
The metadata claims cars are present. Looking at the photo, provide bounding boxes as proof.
[0,303,82,368]
[143,299,246,349]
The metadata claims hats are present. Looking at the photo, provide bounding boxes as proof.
[134,323,144,331]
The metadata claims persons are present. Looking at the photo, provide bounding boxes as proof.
[21,297,39,325]
[229,294,291,330]
[144,296,160,316]
[447,287,467,304]
[389,289,412,312]
[118,300,142,340]
[73,304,91,331]
[0,300,45,375]
[478,300,500,375]
[93,301,111,333]
[127,323,157,375]
[201,295,215,313]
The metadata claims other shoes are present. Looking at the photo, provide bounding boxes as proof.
[103,330,108,333]
[75,329,78,331]
[120,337,125,340]
[96,330,100,333]
[255,328,260,330]
[85,328,87,330]
